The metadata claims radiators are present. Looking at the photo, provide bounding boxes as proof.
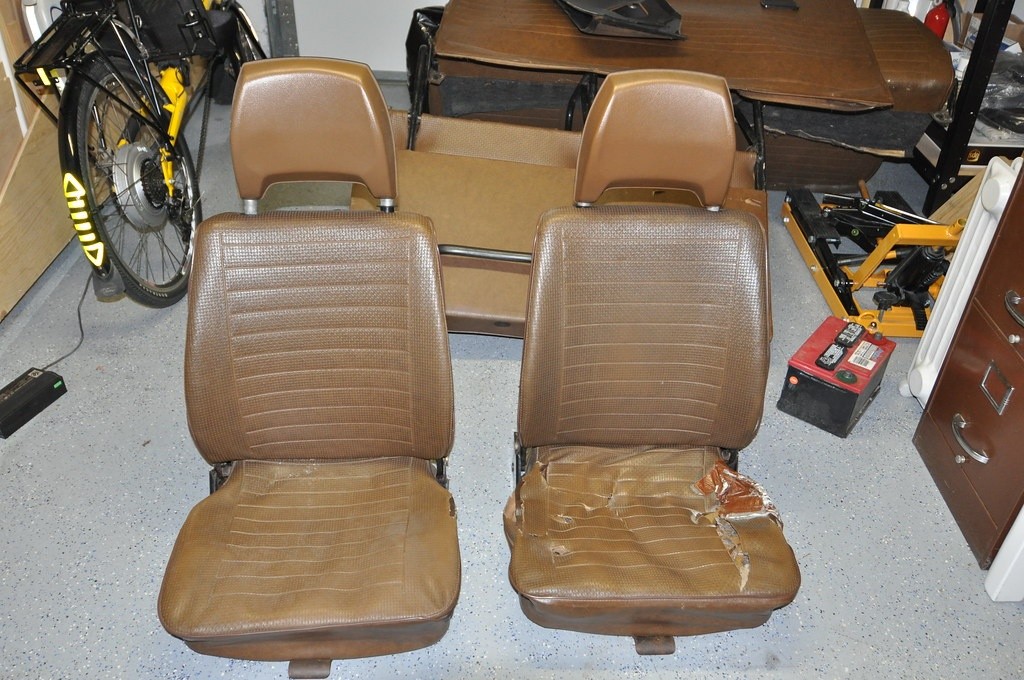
[899,155,1023,411]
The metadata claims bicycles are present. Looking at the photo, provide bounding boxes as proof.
[12,0,267,307]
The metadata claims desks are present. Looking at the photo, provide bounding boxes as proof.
[433,1,894,190]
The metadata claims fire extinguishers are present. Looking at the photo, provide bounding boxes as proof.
[925,0,957,40]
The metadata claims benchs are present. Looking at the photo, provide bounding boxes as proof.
[406,6,954,192]
[389,45,768,340]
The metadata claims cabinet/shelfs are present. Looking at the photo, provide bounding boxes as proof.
[911,158,1024,570]
[868,0,1023,217]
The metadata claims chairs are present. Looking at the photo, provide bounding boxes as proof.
[157,56,461,678]
[503,69,802,655]
